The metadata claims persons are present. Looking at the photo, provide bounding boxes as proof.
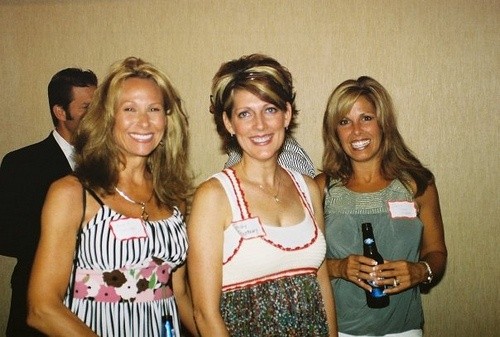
[186,65,338,337]
[210,54,316,178]
[27,57,201,337]
[0,67,98,337]
[314,76,448,337]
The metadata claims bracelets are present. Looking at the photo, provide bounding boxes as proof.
[421,261,433,284]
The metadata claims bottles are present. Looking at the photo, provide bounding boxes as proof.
[359,222,390,308]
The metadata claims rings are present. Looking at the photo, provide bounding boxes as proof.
[393,279,396,286]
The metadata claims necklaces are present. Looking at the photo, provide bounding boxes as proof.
[112,183,154,220]
[258,181,280,201]
